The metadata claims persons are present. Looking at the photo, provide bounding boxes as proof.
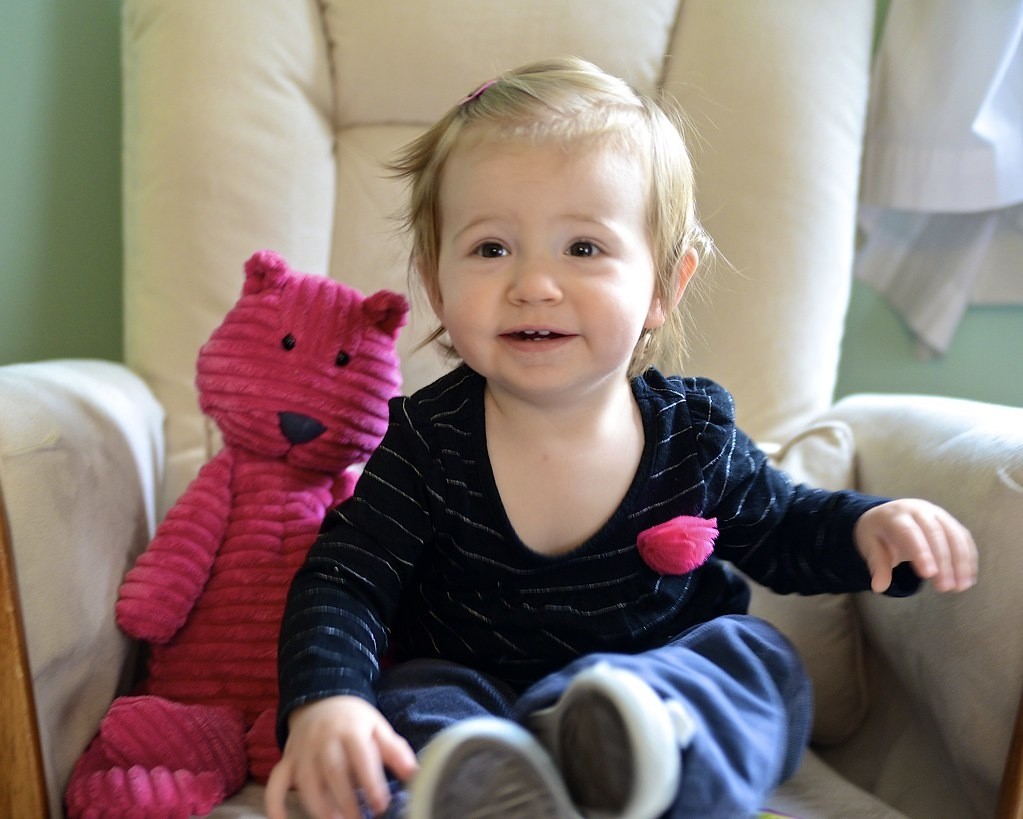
[263,55,979,817]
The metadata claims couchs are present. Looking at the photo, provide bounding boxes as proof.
[1,0,1023,819]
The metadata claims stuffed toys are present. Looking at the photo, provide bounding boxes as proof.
[64,248,411,819]
